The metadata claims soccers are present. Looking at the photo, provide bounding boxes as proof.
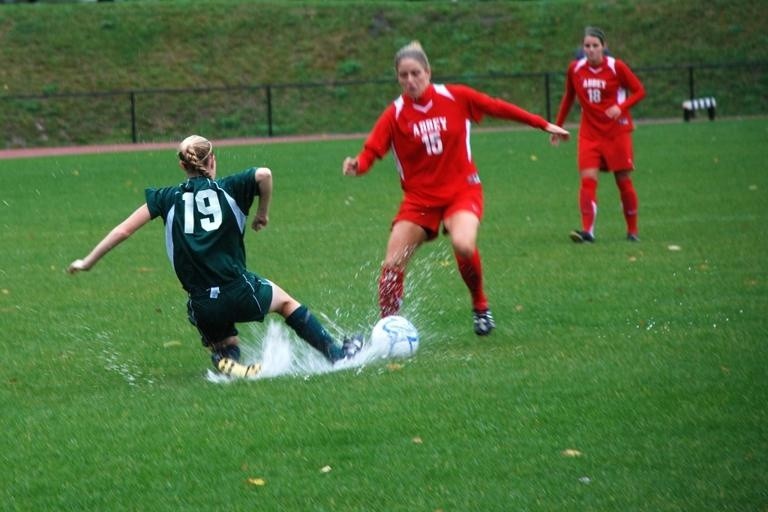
[369,314,419,361]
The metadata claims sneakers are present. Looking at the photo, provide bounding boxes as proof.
[218,358,261,378]
[341,334,362,360]
[474,308,494,336]
[570,231,594,243]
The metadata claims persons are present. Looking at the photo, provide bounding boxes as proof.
[576,27,610,62]
[342,39,572,337]
[551,28,645,244]
[68,136,367,381]
